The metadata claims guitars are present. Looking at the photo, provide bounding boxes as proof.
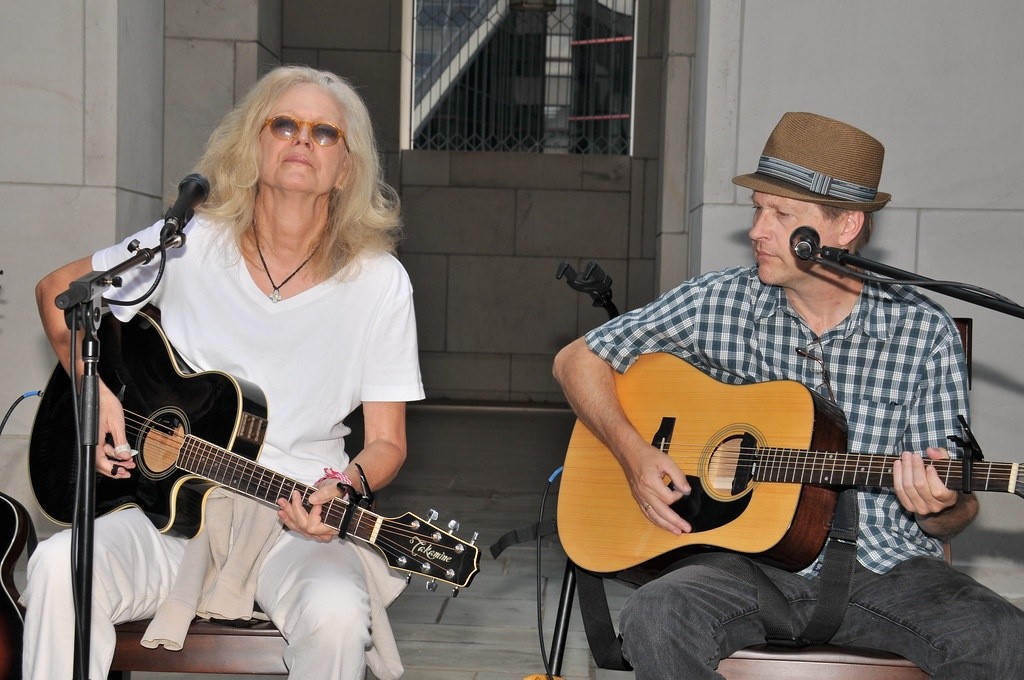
[553,346,1024,590]
[26,305,487,600]
[0,489,37,680]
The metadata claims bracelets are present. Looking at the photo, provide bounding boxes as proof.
[317,468,353,503]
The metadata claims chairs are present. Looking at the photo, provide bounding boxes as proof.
[720,317,973,680]
[103,600,293,680]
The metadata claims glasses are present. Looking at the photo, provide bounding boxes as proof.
[259,116,349,154]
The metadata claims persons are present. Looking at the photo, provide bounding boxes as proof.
[18,65,427,680]
[552,112,1024,680]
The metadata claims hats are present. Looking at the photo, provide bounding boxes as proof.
[733,113,891,212]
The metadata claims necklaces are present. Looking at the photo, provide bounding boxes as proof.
[252,219,318,302]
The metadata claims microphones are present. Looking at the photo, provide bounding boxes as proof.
[158,174,207,243]
[789,226,820,260]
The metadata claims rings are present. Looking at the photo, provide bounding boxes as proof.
[645,505,651,510]
